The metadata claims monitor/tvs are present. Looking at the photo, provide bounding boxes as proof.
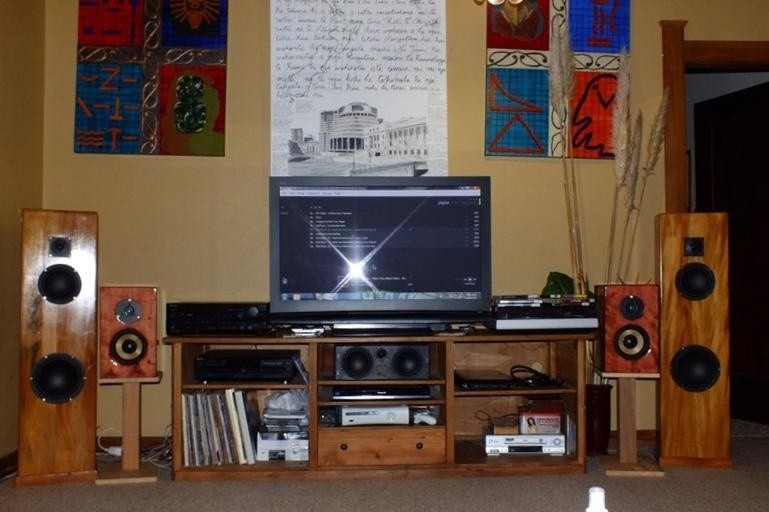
[269,175,492,338]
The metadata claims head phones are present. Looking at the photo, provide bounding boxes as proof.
[510,364,552,388]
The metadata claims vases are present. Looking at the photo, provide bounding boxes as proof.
[588,385,615,455]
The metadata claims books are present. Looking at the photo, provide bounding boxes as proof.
[181,388,308,468]
[489,417,519,435]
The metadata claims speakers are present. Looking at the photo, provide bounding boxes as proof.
[594,283,659,374]
[656,212,736,468]
[14,207,98,486]
[98,285,158,380]
[333,342,433,382]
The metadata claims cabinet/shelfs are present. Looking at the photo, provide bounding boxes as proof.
[164,334,597,481]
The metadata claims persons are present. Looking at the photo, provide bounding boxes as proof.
[527,417,540,433]
